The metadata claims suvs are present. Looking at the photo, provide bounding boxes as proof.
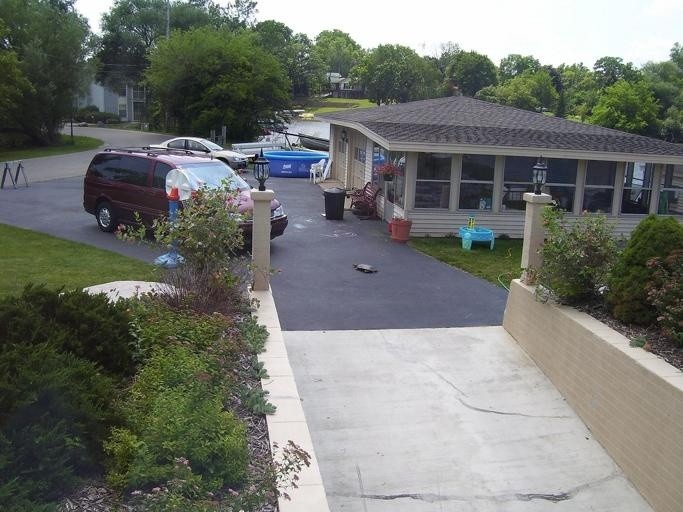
[83,145,291,253]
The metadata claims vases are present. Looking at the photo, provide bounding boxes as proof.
[383,174,393,181]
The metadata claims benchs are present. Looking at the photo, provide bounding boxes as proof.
[351,182,380,220]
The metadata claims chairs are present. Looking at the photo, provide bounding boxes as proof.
[309,158,325,184]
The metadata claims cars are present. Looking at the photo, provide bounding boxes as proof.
[150,138,249,170]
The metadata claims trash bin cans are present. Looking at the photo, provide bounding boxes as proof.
[324,187,347,220]
[141,123,149,132]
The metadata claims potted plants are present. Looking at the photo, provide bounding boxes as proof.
[391,213,413,244]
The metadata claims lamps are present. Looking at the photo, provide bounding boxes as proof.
[342,129,349,144]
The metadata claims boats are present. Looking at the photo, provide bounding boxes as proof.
[256,107,314,137]
[297,127,331,152]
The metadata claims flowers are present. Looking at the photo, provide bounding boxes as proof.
[378,161,395,175]
